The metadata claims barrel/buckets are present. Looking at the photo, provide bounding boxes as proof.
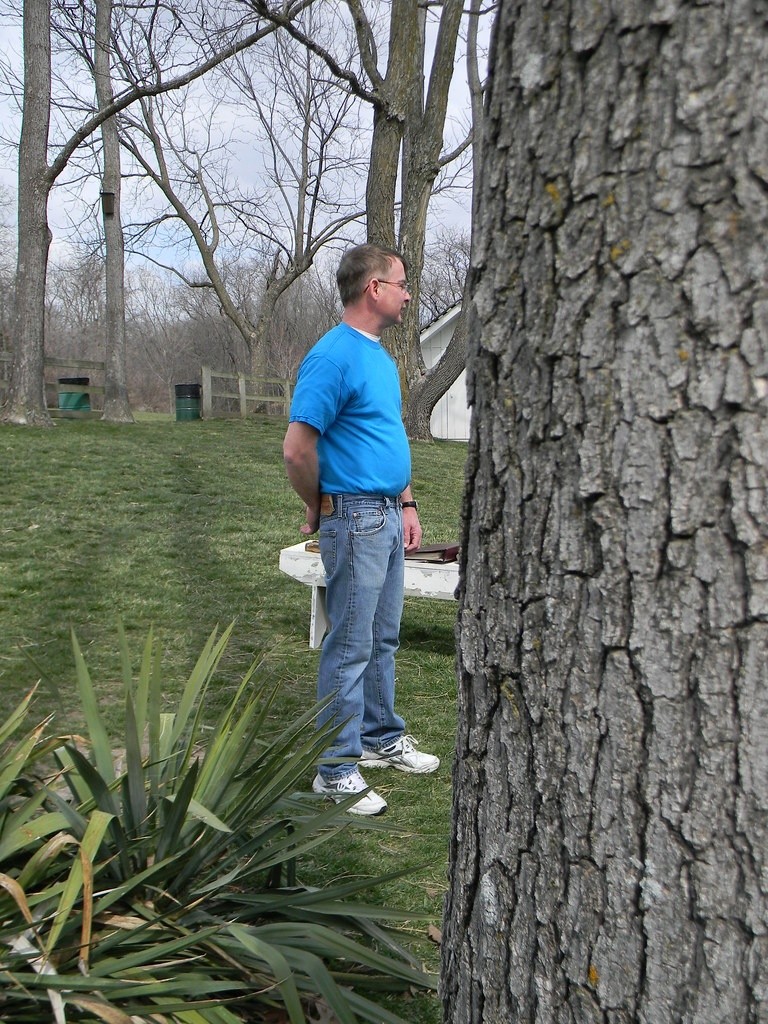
[57,377,92,411]
[175,383,202,422]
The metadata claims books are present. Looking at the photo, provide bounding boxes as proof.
[404,544,460,564]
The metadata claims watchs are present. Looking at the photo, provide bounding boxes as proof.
[399,499,418,512]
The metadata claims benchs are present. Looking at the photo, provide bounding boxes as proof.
[277,539,460,650]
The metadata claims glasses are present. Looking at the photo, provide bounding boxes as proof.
[364,280,408,294]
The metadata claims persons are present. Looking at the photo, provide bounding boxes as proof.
[282,244,441,817]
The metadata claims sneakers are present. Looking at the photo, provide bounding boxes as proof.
[358,735,440,773]
[311,770,389,816]
